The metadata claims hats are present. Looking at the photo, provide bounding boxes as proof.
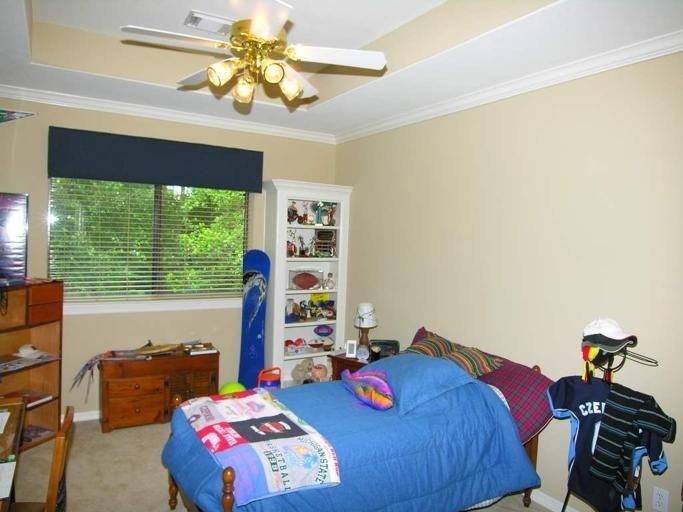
[12,342,48,361]
[579,316,638,356]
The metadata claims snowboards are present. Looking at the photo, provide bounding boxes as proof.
[237,250,269,390]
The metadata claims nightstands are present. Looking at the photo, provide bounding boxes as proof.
[326,352,366,380]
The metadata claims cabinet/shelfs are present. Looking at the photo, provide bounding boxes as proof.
[97,346,219,432]
[264,178,353,385]
[0,278,61,454]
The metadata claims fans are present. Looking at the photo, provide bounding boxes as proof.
[120,0,385,98]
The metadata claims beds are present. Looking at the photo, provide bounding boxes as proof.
[170,326,539,512]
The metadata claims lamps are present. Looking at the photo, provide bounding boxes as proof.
[354,303,379,359]
[207,18,303,104]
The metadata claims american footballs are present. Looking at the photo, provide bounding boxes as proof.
[292,272,319,289]
[314,325,334,336]
[308,337,334,348]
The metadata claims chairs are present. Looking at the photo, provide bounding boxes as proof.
[9,406,73,512]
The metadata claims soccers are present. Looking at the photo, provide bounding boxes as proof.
[322,278,335,289]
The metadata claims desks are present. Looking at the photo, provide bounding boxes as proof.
[0,406,26,512]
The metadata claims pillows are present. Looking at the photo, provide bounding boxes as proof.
[360,335,503,415]
